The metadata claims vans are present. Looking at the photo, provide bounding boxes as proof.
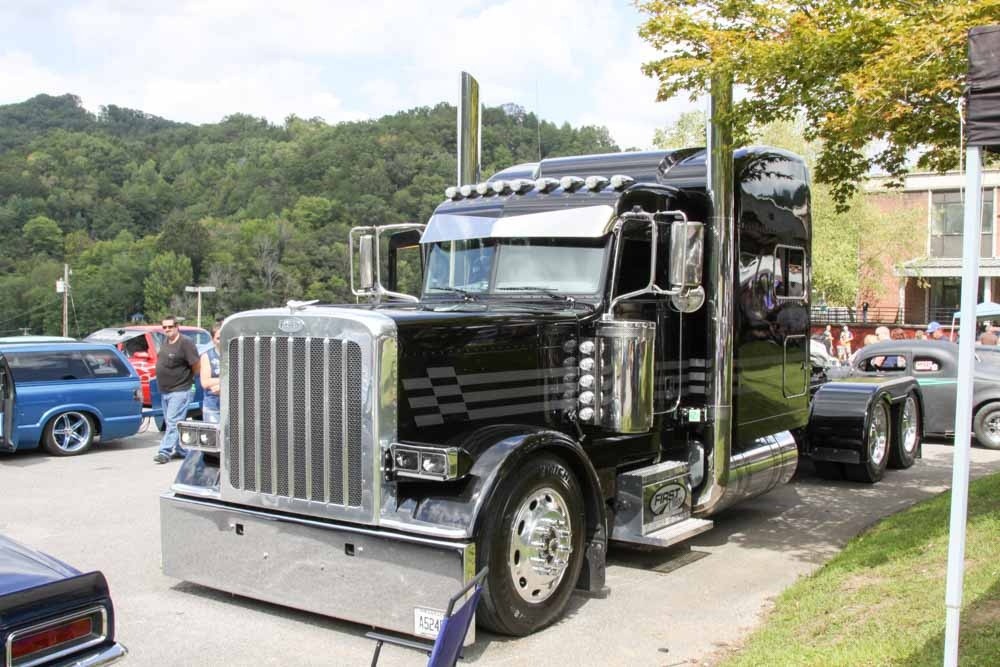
[80,324,214,408]
[0,335,143,456]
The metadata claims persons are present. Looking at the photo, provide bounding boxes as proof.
[153,316,201,462]
[776,282,784,295]
[824,325,834,355]
[864,321,949,370]
[200,321,224,425]
[840,326,853,358]
[981,325,998,345]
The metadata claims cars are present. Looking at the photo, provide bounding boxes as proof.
[0,533,130,667]
[827,339,1000,450]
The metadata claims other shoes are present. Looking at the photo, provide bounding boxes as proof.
[171,451,185,459]
[153,454,168,464]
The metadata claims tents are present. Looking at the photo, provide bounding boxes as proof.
[945,25,1000,667]
[950,301,1000,344]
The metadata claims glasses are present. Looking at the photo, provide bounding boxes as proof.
[163,325,176,329]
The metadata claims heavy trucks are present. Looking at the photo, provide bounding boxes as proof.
[156,143,926,667]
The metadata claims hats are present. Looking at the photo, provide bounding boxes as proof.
[925,321,941,333]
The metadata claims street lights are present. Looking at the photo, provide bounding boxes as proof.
[184,285,216,343]
